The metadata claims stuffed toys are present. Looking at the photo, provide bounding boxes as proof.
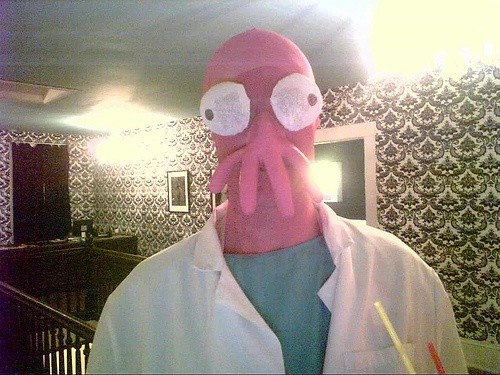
[86,27,468,375]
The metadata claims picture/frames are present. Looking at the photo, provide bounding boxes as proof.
[166,170,190,213]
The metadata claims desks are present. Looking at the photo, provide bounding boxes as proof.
[0,234,140,301]
